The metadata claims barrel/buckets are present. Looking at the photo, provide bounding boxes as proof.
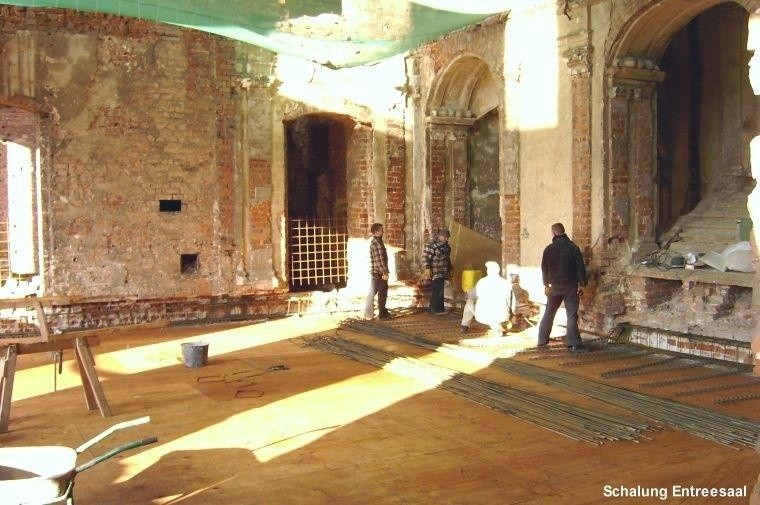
[738,217,753,242]
[461,270,484,293]
[181,342,210,368]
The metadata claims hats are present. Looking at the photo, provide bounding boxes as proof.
[438,229,451,237]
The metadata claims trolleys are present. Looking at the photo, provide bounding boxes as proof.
[0,415,163,505]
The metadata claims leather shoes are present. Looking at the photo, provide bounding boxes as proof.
[570,345,593,353]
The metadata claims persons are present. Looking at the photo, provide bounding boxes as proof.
[460,261,516,336]
[364,223,395,321]
[538,223,588,351]
[424,229,453,315]
